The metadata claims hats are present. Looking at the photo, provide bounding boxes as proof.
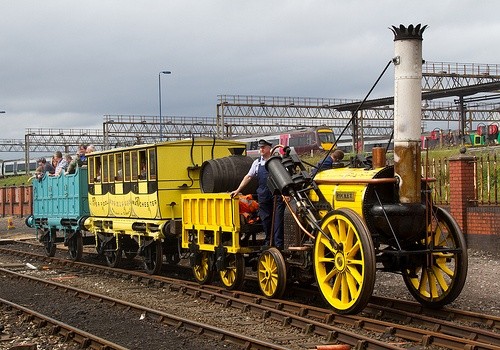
[258,139,273,146]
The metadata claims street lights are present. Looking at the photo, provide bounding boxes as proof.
[157,70,171,143]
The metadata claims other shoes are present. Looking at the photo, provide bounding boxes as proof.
[260,244,284,251]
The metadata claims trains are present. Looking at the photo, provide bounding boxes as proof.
[0,127,337,179]
[25,23,469,317]
[334,135,433,156]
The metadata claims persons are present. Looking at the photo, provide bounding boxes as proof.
[27,143,97,184]
[309,150,346,177]
[229,139,287,251]
